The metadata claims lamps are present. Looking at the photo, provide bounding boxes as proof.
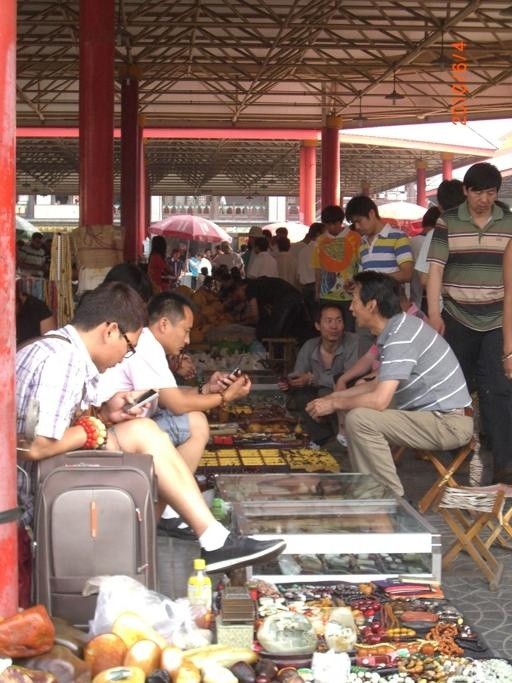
[385,69,405,105]
[353,96,368,126]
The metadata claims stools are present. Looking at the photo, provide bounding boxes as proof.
[416,433,480,514]
[387,444,429,461]
[262,339,297,370]
[433,482,506,592]
[484,483,512,550]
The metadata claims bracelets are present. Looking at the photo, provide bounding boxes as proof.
[501,352,511,363]
[217,390,228,410]
[279,582,511,683]
[75,414,108,452]
[199,382,205,397]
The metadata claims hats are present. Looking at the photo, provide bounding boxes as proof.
[244,227,266,237]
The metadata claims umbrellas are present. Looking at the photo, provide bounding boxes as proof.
[149,214,233,254]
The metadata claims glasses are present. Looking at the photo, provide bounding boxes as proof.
[107,321,136,359]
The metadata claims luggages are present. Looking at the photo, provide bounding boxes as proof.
[25,452,159,627]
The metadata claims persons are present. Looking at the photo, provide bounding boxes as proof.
[15,232,47,276]
[409,206,442,316]
[278,304,374,441]
[304,271,473,498]
[99,295,250,541]
[415,179,465,285]
[500,238,511,380]
[312,205,360,303]
[13,287,56,352]
[345,194,413,298]
[335,283,426,445]
[146,237,246,296]
[248,223,322,283]
[425,162,510,376]
[18,281,287,573]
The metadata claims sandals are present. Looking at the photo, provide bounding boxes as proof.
[156,514,200,540]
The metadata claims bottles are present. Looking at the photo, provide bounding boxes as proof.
[209,498,225,527]
[188,560,211,629]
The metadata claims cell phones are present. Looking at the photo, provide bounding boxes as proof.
[221,367,242,386]
[292,376,298,380]
[121,389,160,414]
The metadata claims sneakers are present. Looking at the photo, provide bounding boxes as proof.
[196,529,288,574]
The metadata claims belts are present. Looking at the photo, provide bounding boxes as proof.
[461,407,475,418]
